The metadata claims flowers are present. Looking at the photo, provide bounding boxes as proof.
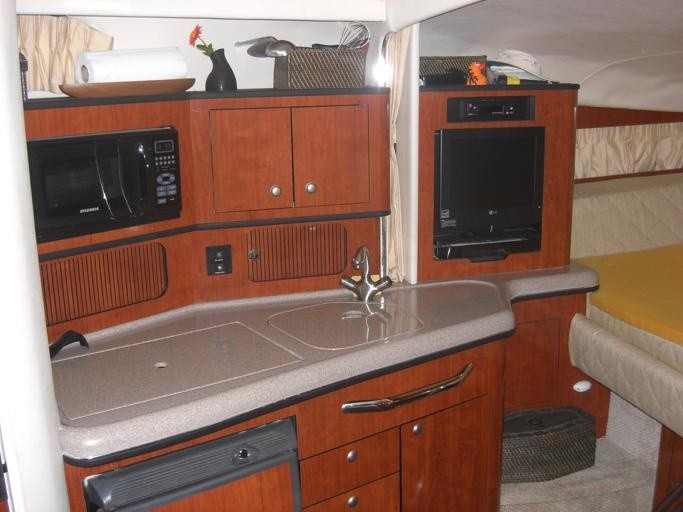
[189,24,213,56]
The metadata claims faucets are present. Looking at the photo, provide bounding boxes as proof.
[339,300,393,340]
[339,246,393,300]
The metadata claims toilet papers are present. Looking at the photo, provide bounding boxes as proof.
[75,48,189,82]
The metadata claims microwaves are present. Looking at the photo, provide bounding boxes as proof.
[26,125,181,244]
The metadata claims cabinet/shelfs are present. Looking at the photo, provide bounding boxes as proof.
[296,339,506,512]
[190,94,390,223]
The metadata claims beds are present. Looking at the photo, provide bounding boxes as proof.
[570,168,683,512]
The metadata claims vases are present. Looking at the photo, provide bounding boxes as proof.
[205,49,237,92]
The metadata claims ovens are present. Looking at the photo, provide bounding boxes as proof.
[433,126,546,263]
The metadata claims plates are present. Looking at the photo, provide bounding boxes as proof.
[58,78,195,98]
